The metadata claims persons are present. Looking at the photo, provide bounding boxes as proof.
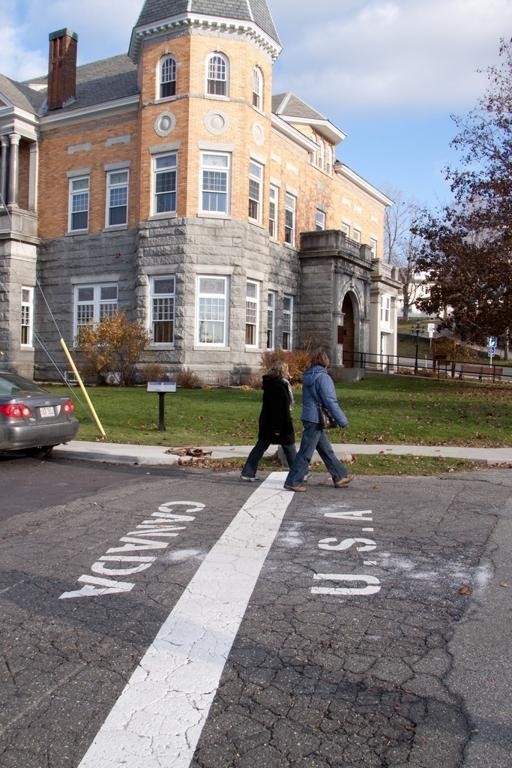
[240,359,313,483]
[283,351,355,492]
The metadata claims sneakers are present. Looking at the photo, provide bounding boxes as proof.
[240,474,261,482]
[334,473,356,488]
[284,482,307,492]
[302,470,312,481]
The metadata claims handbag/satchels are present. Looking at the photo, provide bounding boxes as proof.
[318,406,337,429]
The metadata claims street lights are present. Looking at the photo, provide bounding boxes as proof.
[410,319,424,374]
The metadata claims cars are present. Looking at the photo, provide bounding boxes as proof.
[0,370,80,461]
